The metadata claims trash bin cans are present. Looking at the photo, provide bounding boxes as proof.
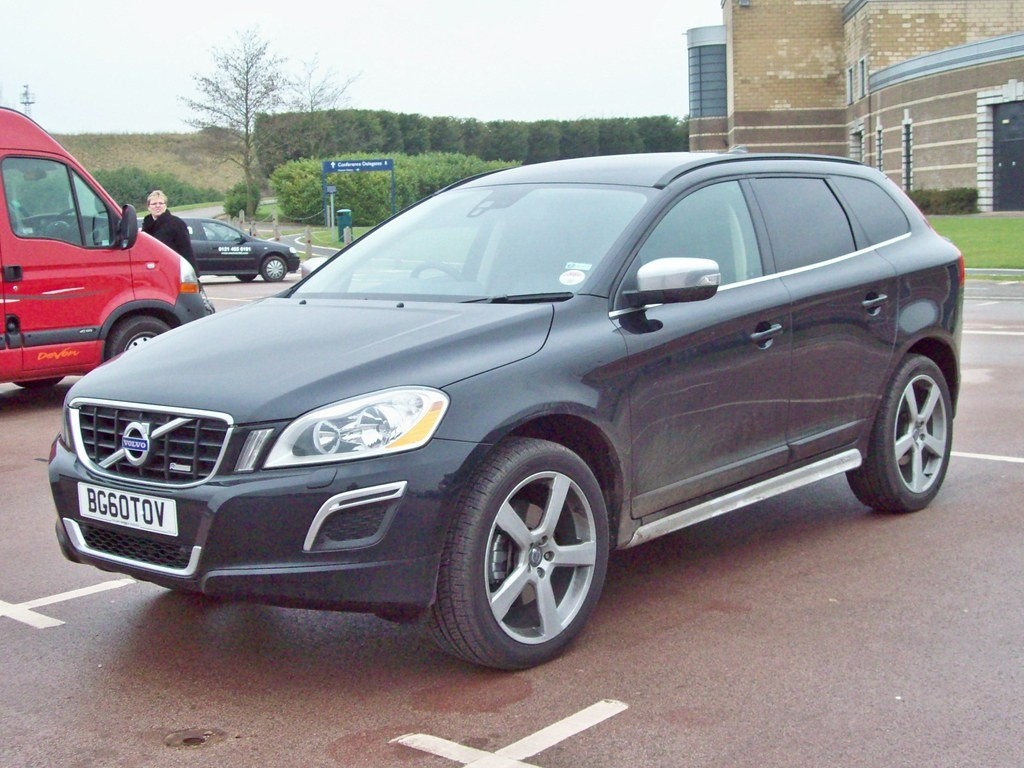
[336,209,353,239]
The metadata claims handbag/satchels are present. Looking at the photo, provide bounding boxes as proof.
[198,279,215,315]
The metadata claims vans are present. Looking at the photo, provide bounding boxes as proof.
[0,104,217,387]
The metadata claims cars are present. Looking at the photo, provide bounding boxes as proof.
[135,216,302,283]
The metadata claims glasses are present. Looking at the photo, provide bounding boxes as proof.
[149,202,166,206]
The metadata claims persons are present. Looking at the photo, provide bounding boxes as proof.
[141,190,201,289]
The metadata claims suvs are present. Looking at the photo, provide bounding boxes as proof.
[46,146,967,674]
